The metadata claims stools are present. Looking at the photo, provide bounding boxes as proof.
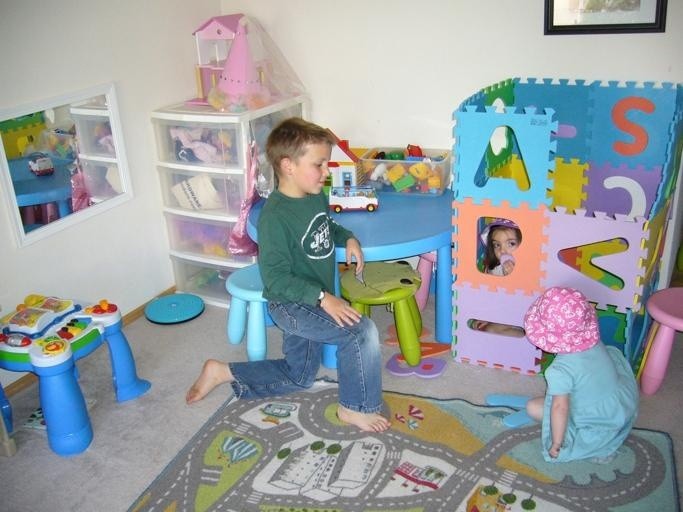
[640,287,683,396]
[225,252,437,367]
[24,203,56,223]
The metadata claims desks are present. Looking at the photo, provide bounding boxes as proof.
[246,188,453,370]
[7,155,73,218]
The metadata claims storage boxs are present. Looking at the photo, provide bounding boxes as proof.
[69,102,118,204]
[359,145,451,198]
[150,94,309,312]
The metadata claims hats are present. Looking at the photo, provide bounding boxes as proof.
[479,218,520,248]
[524,287,600,354]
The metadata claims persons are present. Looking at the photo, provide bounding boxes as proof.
[469,219,525,336]
[185,116,392,434]
[522,287,638,463]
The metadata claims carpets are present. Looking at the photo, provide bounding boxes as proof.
[126,376,681,512]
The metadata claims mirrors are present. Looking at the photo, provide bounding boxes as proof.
[0,82,135,250]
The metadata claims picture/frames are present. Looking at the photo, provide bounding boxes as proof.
[542,0,668,35]
